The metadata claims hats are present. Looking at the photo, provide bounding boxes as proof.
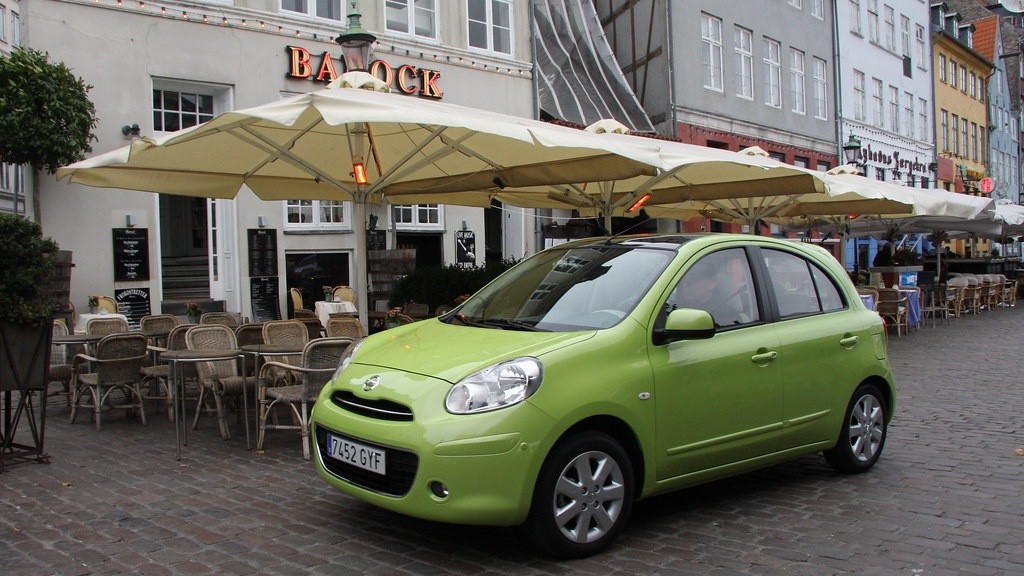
[682,260,718,281]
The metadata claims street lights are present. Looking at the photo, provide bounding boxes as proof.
[337,1,378,337]
[843,131,862,283]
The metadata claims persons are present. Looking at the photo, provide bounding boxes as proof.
[954,248,989,259]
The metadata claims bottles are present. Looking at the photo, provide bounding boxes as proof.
[325,288,340,302]
[99,306,108,315]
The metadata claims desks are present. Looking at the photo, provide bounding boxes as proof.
[859,295,877,312]
[52,329,170,423]
[329,311,437,330]
[314,300,358,338]
[159,350,251,461]
[898,289,922,333]
[74,313,129,355]
[240,344,304,447]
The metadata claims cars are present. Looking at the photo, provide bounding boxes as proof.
[308,232,900,555]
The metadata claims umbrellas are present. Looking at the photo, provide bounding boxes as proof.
[490,120,1023,284]
[54,70,674,340]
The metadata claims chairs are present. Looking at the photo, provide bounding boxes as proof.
[856,271,1019,338]
[716,271,751,324]
[403,304,429,324]
[331,286,357,305]
[290,288,303,311]
[435,304,455,316]
[385,313,414,329]
[48,294,363,462]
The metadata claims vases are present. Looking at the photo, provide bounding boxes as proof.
[325,294,333,302]
[91,306,97,313]
[188,314,201,324]
[386,322,401,328]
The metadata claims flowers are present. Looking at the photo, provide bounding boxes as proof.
[87,295,99,307]
[184,301,203,317]
[385,307,402,324]
[323,287,333,296]
